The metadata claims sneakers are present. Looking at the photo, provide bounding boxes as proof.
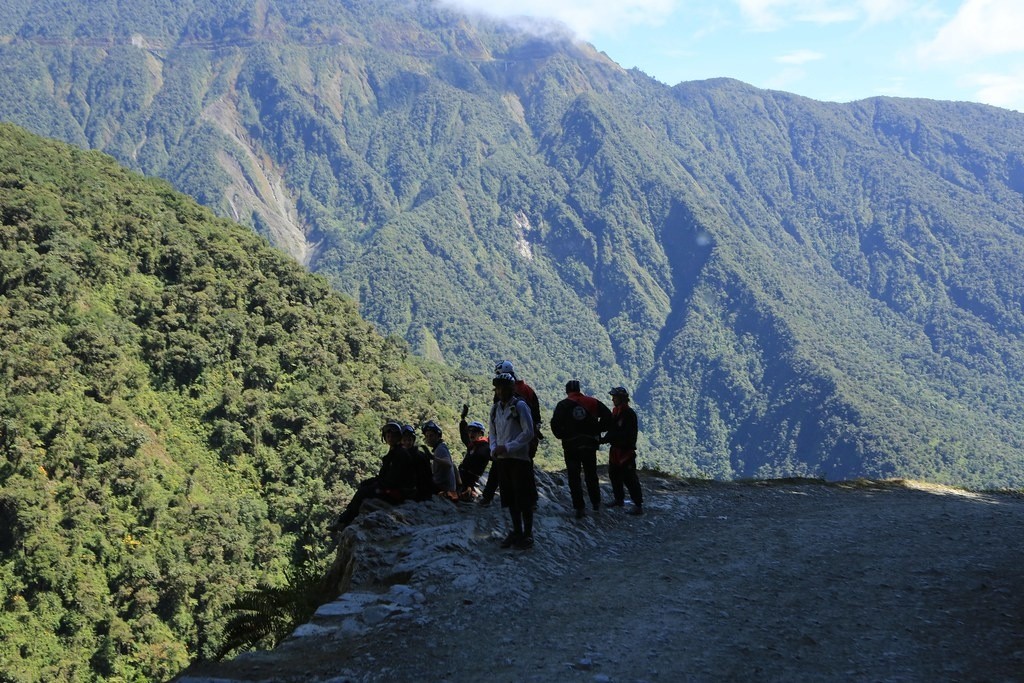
[477,498,491,506]
[499,530,523,549]
[325,524,345,532]
[624,505,644,515]
[531,497,539,510]
[574,506,585,516]
[590,506,599,512]
[604,499,625,507]
[511,533,536,550]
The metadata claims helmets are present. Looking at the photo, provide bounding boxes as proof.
[494,361,514,375]
[422,422,443,438]
[608,386,629,398]
[400,424,416,439]
[468,421,485,436]
[382,422,402,437]
[492,373,515,386]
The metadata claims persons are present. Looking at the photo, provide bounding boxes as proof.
[488,373,536,549]
[422,421,456,490]
[600,386,643,514]
[326,423,411,531]
[480,360,544,505]
[551,380,613,518]
[458,403,490,499]
[400,426,430,501]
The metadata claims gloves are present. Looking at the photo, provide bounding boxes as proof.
[460,404,468,420]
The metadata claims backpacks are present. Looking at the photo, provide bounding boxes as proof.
[493,398,544,458]
